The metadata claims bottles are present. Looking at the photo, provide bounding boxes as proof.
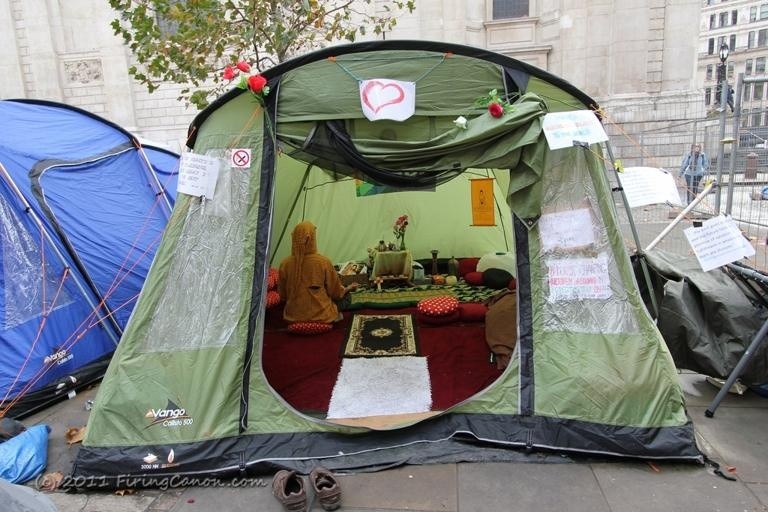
[447,256,460,282]
[379,240,385,253]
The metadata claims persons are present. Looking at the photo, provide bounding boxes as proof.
[276,221,357,324]
[679,143,711,206]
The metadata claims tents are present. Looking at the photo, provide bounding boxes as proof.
[1,99,185,422]
[53,37,707,496]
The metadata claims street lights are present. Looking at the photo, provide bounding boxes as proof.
[714,43,729,84]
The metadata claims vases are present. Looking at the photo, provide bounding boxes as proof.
[400,237,405,250]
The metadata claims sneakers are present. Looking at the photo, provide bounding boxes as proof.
[309,466,342,511]
[271,470,307,512]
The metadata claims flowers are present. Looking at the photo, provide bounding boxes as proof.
[393,215,409,239]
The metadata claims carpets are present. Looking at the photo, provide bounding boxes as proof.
[325,314,434,419]
